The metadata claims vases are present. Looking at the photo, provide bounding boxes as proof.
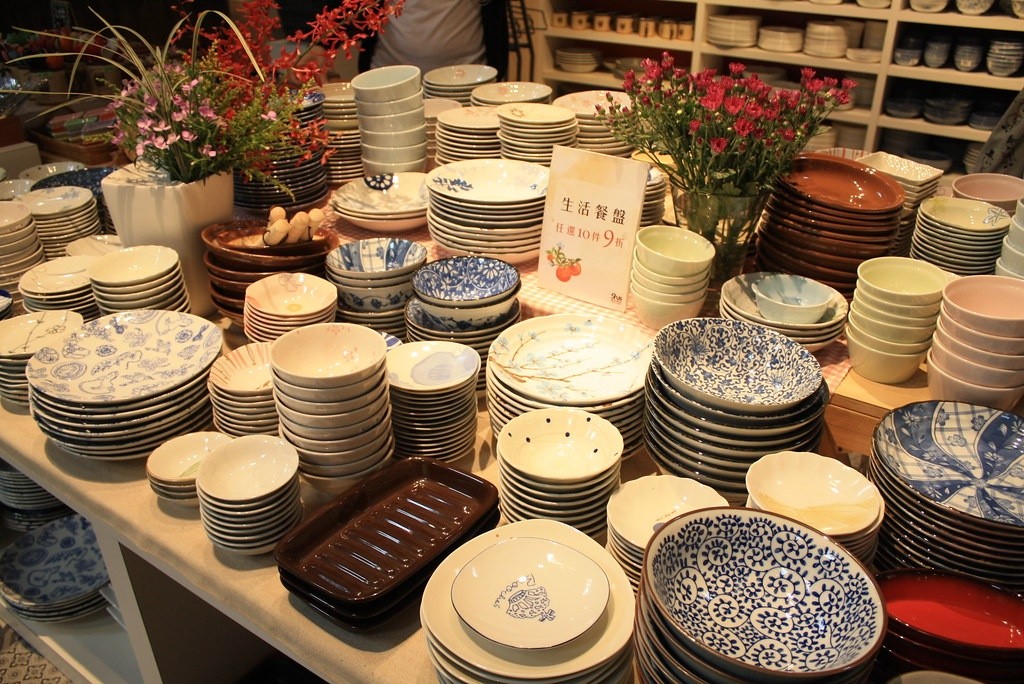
[668,169,772,317]
[100,163,235,316]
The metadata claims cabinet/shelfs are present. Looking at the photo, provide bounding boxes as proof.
[538,0,1024,177]
[0,183,1024,684]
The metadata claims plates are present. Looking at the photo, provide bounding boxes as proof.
[1,16,1024,684]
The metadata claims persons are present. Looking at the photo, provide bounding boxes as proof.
[359,0,509,83]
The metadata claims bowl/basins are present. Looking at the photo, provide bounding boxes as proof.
[352,66,428,177]
[629,224,716,330]
[845,197,1024,414]
[752,276,832,324]
[272,324,396,496]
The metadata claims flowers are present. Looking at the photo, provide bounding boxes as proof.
[0,0,408,202]
[593,50,859,281]
